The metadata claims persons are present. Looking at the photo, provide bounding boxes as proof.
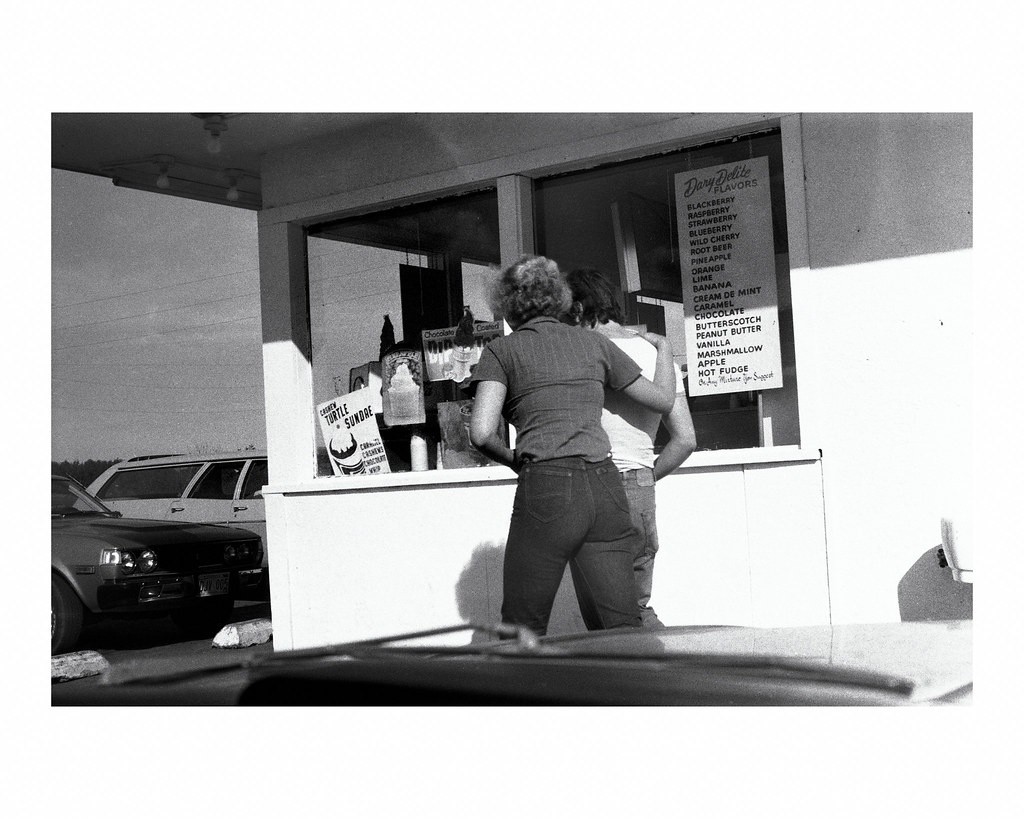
[470,253,676,640]
[563,267,699,629]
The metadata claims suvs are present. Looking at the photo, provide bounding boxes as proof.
[72,452,269,568]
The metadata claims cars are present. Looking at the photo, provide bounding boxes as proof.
[51,473,264,655]
